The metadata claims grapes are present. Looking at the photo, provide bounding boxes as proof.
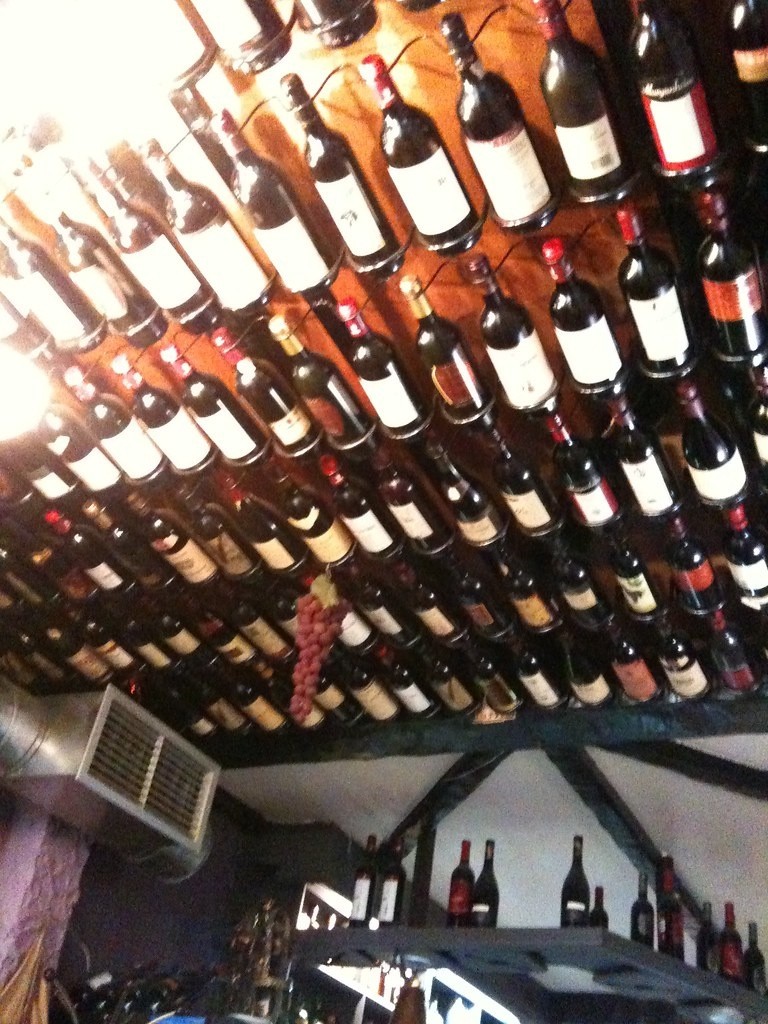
[289,592,347,723]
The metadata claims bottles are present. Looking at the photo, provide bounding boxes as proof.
[377,836,405,927]
[40,890,341,1024]
[719,903,746,981]
[655,854,684,959]
[0,1,768,761]
[349,835,379,929]
[446,839,474,926]
[591,886,609,928]
[561,835,590,927]
[697,903,719,973]
[470,840,499,927]
[745,922,767,995]
[631,872,654,947]
